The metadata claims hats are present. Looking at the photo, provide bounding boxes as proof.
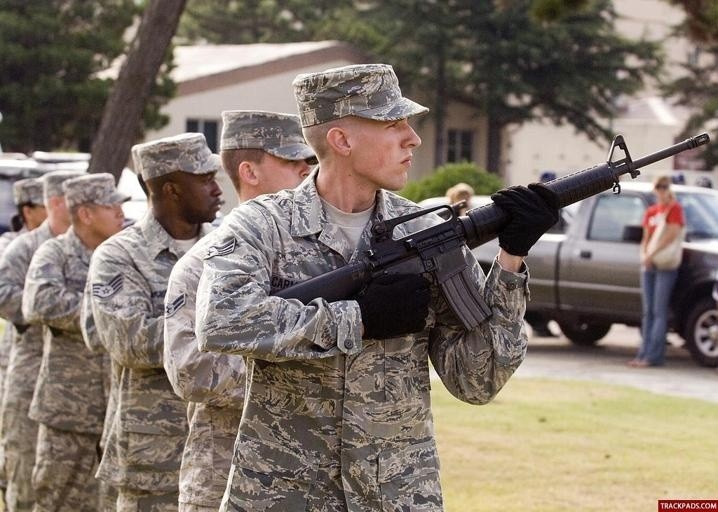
[12,169,132,208]
[220,110,320,164]
[131,132,219,183]
[292,63,430,129]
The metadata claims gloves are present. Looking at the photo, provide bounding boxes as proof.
[491,183,560,256]
[355,274,431,340]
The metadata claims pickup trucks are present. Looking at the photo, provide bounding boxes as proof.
[467,182,718,367]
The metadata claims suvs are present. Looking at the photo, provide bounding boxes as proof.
[0,154,223,239]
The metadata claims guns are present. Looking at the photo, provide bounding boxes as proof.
[255,134,710,369]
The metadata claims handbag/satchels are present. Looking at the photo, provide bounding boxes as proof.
[646,224,686,272]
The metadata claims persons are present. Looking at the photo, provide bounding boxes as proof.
[626,176,685,368]
[447,183,475,217]
[531,172,558,337]
[195,62,560,511]
[0,111,319,512]
